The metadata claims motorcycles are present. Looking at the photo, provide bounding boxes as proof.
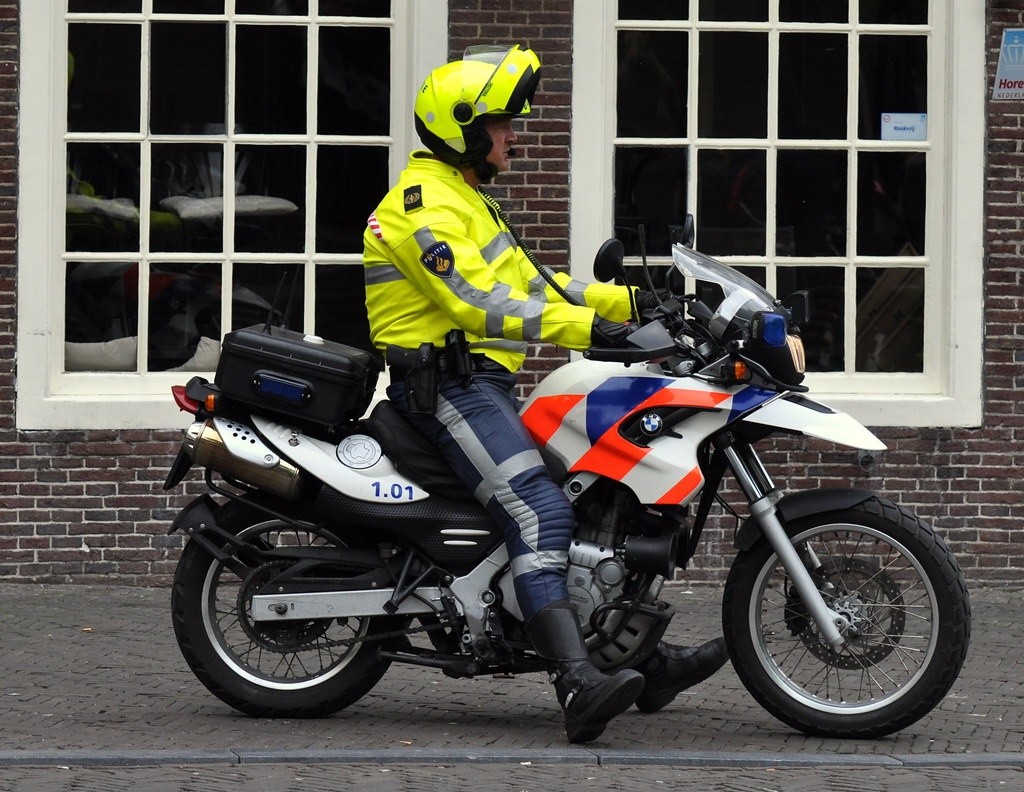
[66,194,298,371]
[162,217,973,737]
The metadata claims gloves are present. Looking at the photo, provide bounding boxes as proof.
[594,317,631,367]
[639,289,675,307]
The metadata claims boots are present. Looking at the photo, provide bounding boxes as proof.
[524,602,643,743]
[632,636,728,712]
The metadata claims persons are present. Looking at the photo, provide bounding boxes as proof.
[361,43,730,743]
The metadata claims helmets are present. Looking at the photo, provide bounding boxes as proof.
[415,44,543,164]
